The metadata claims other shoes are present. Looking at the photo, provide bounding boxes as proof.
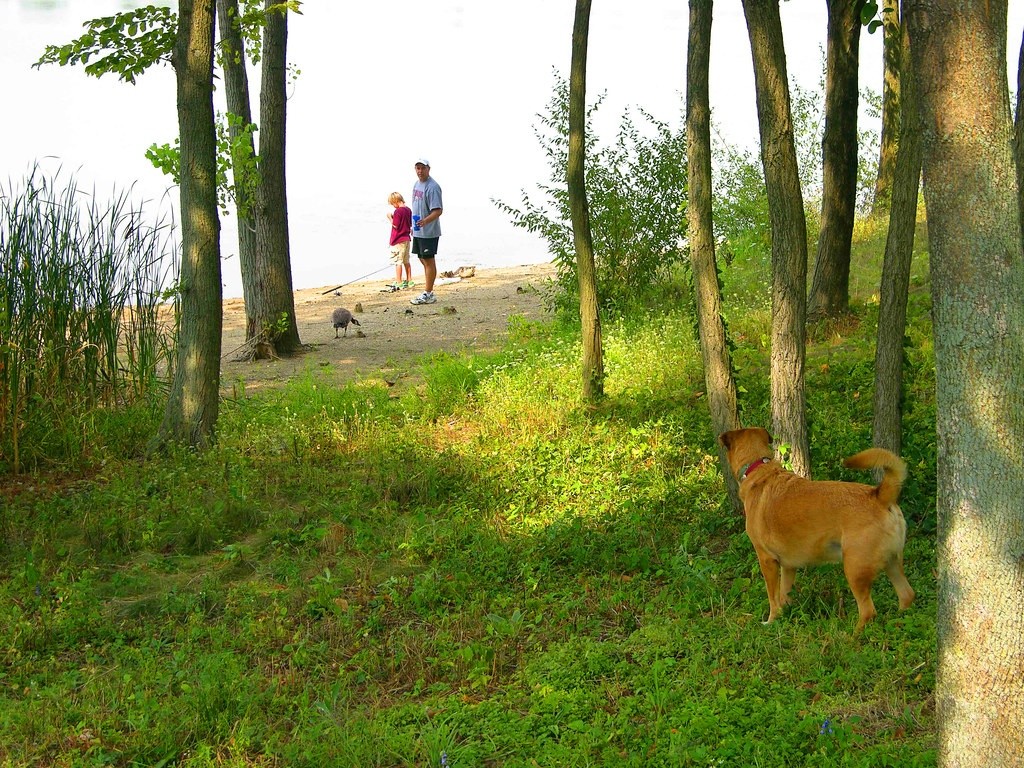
[401,280,415,288]
[410,290,437,304]
[390,281,404,290]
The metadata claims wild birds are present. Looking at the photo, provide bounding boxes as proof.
[330,308,362,340]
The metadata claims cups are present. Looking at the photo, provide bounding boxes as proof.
[412,215,420,231]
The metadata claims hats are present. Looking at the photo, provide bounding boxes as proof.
[414,158,431,168]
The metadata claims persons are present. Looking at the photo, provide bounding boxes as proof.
[410,158,443,305]
[386,192,414,293]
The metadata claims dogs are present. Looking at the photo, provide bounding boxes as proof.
[719,427,915,644]
